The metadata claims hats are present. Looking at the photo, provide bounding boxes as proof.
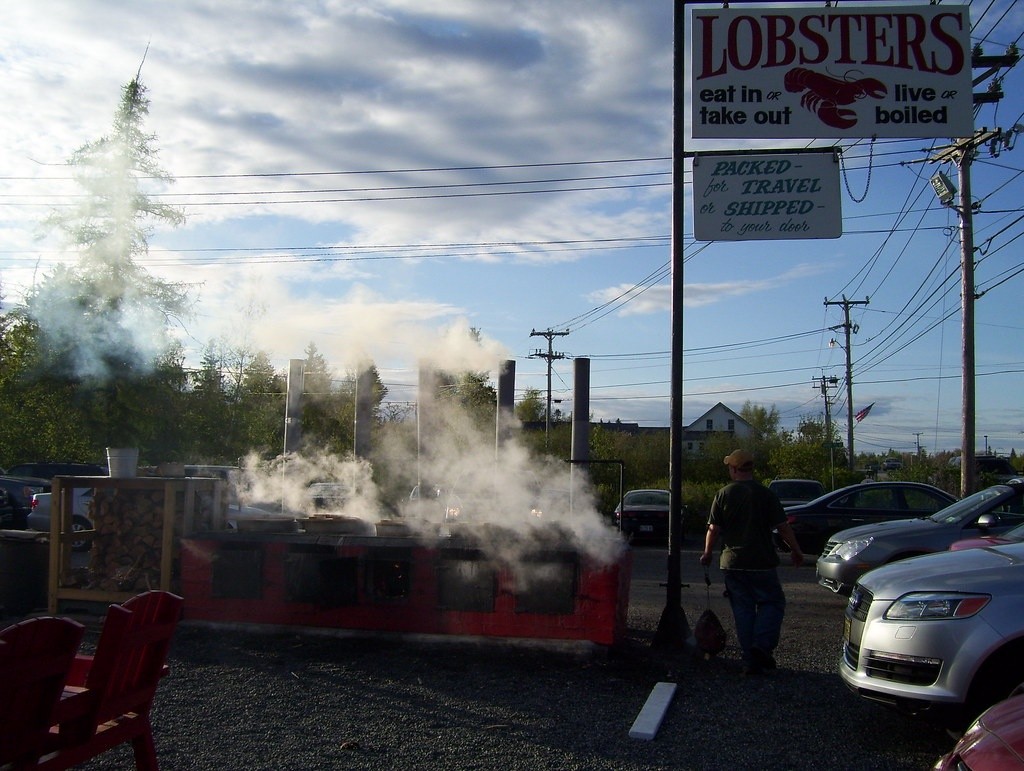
[723,449,757,468]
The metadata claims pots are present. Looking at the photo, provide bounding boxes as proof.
[375,524,409,536]
[303,520,361,533]
[236,520,298,534]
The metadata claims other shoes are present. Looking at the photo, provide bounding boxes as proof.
[752,638,777,670]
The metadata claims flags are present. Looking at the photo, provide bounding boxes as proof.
[855,402,874,422]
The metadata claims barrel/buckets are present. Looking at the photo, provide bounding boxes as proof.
[106,446,138,478]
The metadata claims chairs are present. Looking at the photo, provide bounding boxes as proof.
[0,590,186,771]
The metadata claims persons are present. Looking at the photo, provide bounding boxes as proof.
[700,449,804,678]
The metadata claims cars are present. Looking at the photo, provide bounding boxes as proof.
[768,479,826,512]
[929,695,1024,771]
[25,487,96,552]
[772,481,1024,555]
[838,541,1024,722]
[947,524,1024,556]
[304,483,351,513]
[814,478,1024,599]
[530,494,573,526]
[443,470,541,527]
[5,462,111,479]
[613,488,688,538]
[409,484,435,508]
[0,474,54,532]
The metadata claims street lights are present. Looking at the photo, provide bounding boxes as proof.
[983,435,990,455]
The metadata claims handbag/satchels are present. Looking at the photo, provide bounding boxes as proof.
[695,562,727,656]
